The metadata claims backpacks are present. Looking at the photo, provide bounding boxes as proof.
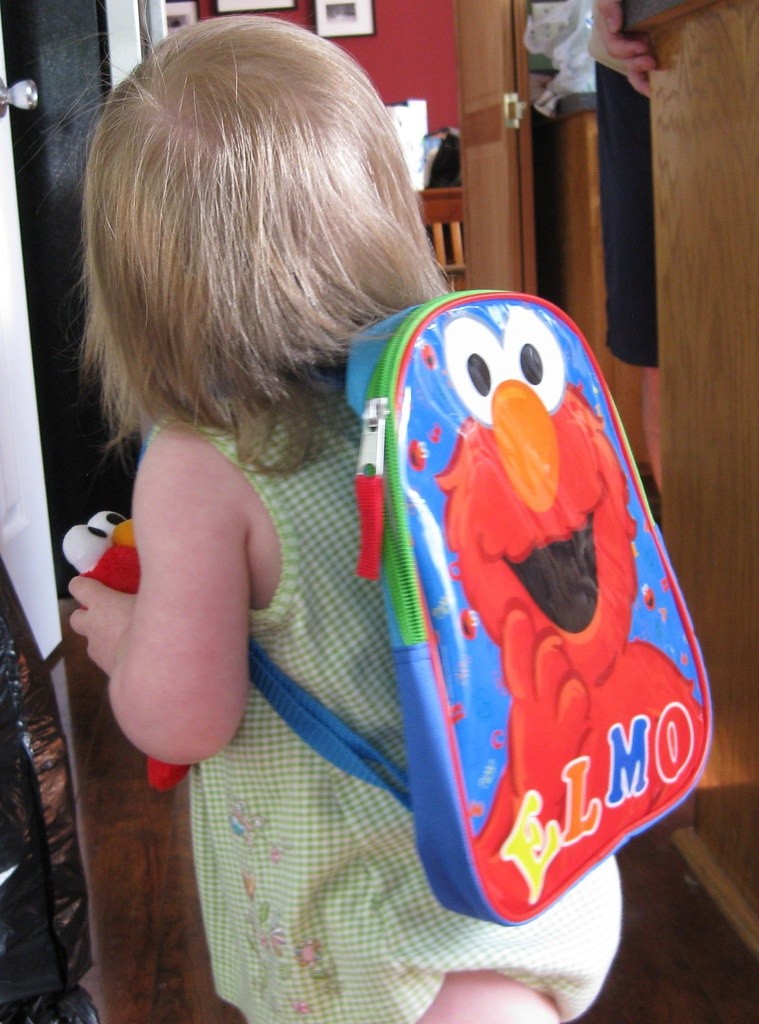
[136,287,714,930]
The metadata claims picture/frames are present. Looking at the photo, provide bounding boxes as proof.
[313,0,375,39]
[165,0,200,34]
[214,0,296,16]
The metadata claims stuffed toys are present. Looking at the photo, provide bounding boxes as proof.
[63,508,195,793]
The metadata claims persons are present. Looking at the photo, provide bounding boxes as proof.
[591,0,668,504]
[68,16,625,1024]
